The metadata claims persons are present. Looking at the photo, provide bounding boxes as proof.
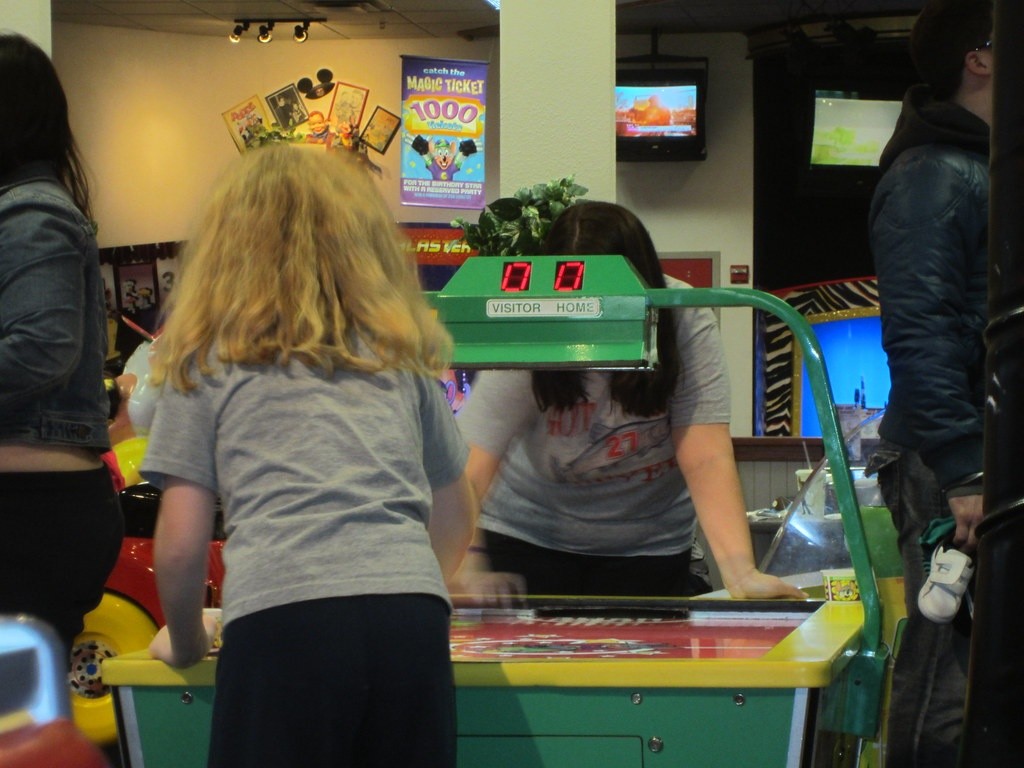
[866,1,997,768]
[0,28,127,714]
[452,198,807,608]
[141,145,481,768]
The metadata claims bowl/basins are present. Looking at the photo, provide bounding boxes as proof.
[820,568,861,604]
[825,467,879,509]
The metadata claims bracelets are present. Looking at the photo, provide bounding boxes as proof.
[467,545,486,553]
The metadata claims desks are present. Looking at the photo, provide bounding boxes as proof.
[746,507,845,536]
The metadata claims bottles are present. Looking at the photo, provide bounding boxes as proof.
[836,403,862,462]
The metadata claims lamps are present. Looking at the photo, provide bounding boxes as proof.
[293,21,311,43]
[229,22,250,44]
[257,20,275,43]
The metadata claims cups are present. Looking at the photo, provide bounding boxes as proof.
[203,607,221,626]
[795,469,826,518]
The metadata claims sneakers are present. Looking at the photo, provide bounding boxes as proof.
[918,539,974,623]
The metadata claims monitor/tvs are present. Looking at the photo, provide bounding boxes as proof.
[615,66,707,162]
[803,86,905,173]
